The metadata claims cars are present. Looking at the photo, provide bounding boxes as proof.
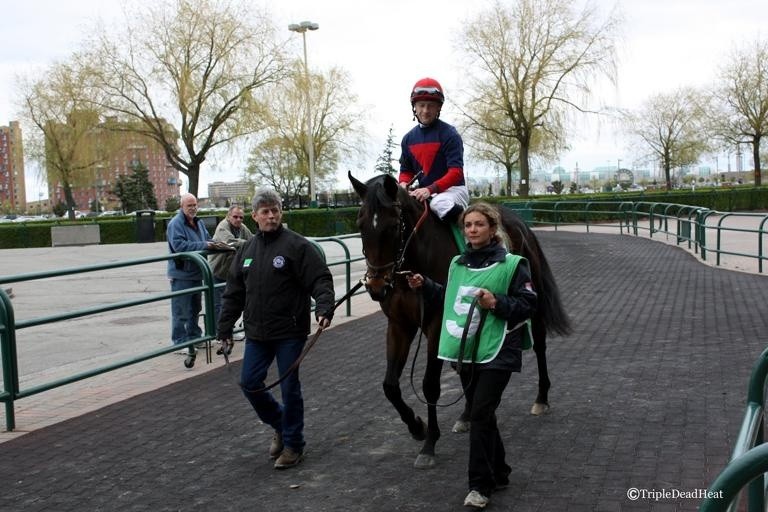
[0,207,231,223]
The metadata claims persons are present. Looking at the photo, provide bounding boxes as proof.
[397,77,469,243]
[207,206,254,332]
[407,202,537,507]
[216,190,335,468]
[166,194,213,354]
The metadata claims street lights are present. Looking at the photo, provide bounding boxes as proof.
[616,158,623,185]
[92,163,104,214]
[288,19,321,208]
[125,144,147,164]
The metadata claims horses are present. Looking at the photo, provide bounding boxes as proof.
[347,169,573,469]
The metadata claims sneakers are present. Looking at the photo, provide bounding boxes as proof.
[174,347,198,355]
[232,333,245,341]
[195,340,218,348]
[274,448,305,469]
[464,490,490,508]
[270,431,284,458]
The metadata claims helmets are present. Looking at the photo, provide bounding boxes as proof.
[410,78,445,104]
